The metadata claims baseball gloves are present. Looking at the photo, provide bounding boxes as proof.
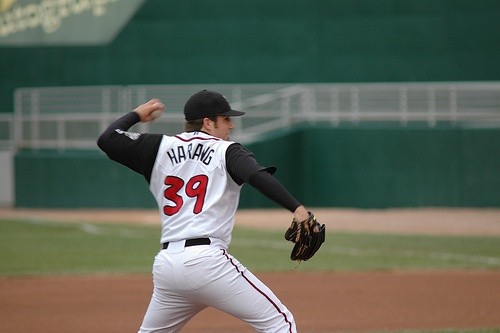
[285,211,326,262]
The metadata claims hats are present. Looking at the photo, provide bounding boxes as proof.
[183,89,246,121]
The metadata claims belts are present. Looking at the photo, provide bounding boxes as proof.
[162,238,211,249]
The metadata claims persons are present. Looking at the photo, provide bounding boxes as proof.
[97,89,325,333]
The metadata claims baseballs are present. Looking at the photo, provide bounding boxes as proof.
[151,102,166,117]
